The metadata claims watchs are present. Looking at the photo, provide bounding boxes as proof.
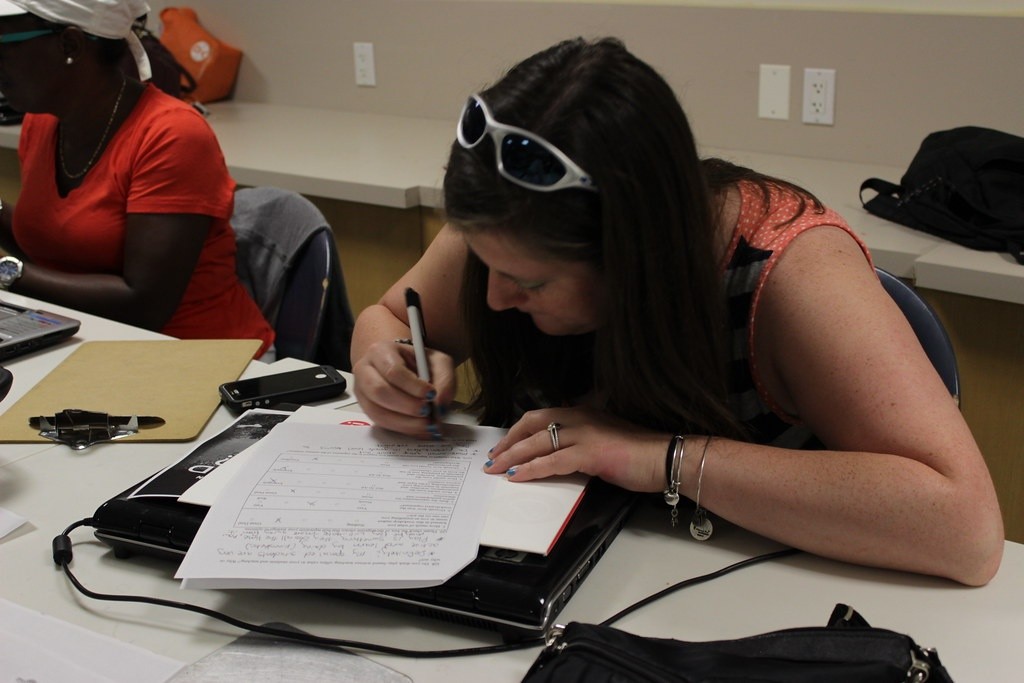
[0,256,24,291]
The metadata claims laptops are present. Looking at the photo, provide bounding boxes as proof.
[0,300,82,363]
[93,401,638,635]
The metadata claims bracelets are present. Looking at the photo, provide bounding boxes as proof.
[697,434,712,506]
[664,435,684,527]
[394,337,414,346]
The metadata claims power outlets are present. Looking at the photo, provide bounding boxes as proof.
[353,42,376,88]
[802,68,836,128]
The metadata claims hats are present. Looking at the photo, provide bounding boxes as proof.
[0,0,152,81]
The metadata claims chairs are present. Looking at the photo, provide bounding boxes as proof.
[225,186,357,372]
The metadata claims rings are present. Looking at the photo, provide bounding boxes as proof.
[547,421,562,450]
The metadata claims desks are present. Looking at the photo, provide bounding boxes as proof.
[0,97,461,211]
[0,289,1024,683]
[912,240,1024,305]
[417,143,946,280]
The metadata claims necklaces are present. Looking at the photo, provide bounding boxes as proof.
[59,76,125,178]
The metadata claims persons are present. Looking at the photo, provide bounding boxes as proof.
[352,36,1005,589]
[0,3,277,364]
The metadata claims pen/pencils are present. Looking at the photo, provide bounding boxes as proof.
[29,409,167,428]
[405,287,442,442]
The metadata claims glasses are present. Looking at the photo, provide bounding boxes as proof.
[456,92,594,194]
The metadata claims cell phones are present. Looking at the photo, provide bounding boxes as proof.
[219,364,348,411]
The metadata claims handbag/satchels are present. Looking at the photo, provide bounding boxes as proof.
[858,127,1024,268]
[523,601,951,683]
